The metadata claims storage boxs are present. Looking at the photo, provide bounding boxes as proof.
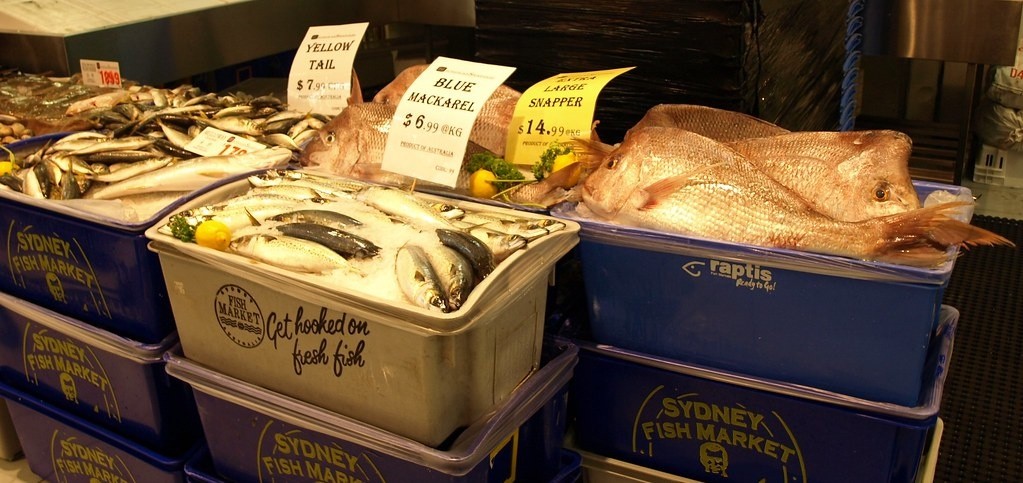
[0,293,203,459]
[0,76,265,345]
[143,172,582,447]
[162,335,580,483]
[563,425,704,483]
[0,381,205,483]
[550,179,974,410]
[553,303,962,483]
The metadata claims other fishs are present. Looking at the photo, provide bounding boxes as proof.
[0,64,1017,314]
